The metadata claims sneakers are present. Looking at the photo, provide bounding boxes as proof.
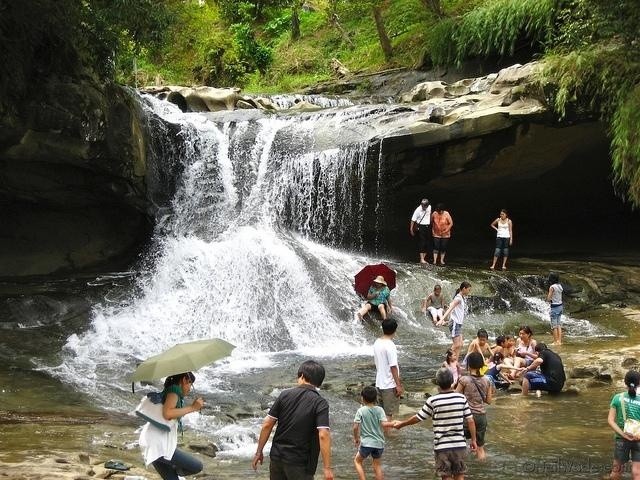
[104,460,130,471]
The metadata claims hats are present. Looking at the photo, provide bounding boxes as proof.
[373,275,388,285]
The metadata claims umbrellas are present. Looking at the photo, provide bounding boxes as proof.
[128,338,236,414]
[354,262,397,301]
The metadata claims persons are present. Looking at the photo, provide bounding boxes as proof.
[489,209,514,271]
[430,203,454,263]
[607,370,640,480]
[138,371,203,479]
[409,197,432,264]
[356,275,391,323]
[252,360,334,480]
[373,317,404,439]
[394,271,566,480]
[352,386,398,479]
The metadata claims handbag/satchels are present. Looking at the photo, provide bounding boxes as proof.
[135,392,178,430]
[623,417,640,440]
[413,222,420,232]
